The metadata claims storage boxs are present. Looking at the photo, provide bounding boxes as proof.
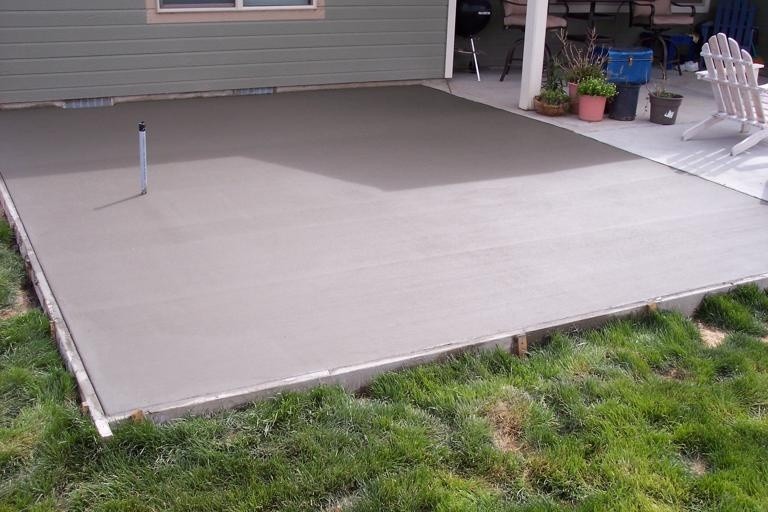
[596,43,653,85]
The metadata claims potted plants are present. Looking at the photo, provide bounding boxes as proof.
[643,36,684,125]
[533,84,570,117]
[552,25,611,116]
[575,78,617,123]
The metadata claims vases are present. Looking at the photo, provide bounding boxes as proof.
[608,85,640,121]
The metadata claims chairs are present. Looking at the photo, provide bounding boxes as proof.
[628,0,697,80]
[680,32,768,157]
[500,0,570,82]
[455,1,493,82]
[700,0,758,73]
